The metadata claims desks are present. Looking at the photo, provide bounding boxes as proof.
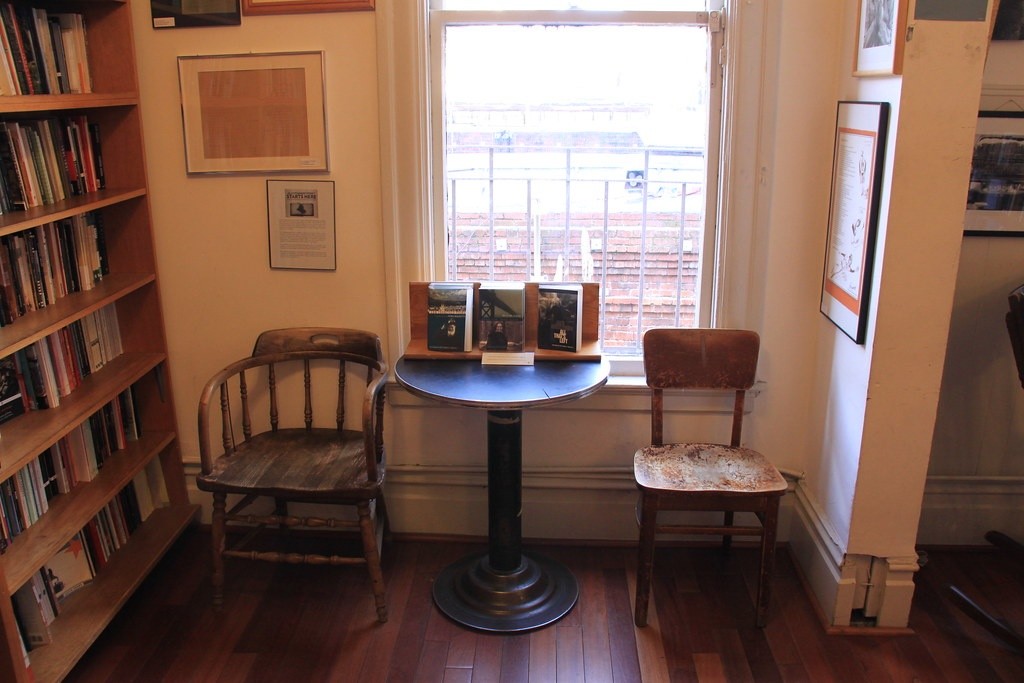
[393,355,607,635]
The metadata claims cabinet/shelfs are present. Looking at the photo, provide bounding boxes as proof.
[0,0,202,682]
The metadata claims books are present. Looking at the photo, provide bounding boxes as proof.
[537,283,583,353]
[426,282,474,352]
[0,0,174,682]
[477,281,525,352]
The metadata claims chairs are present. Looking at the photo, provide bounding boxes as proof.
[947,287,1024,653]
[634,328,787,633]
[195,326,392,621]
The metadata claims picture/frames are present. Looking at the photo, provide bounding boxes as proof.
[962,111,1024,237]
[175,49,330,175]
[851,0,909,77]
[820,100,888,344]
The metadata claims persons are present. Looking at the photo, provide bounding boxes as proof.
[445,318,457,337]
[483,321,509,352]
